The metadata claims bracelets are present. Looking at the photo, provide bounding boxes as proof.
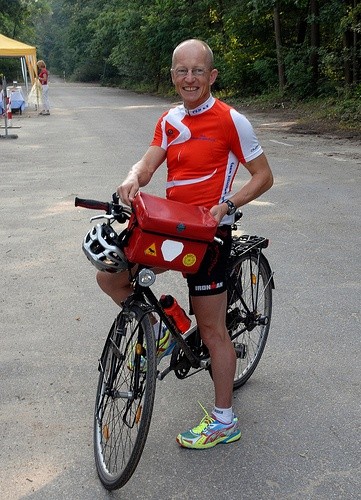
[39,77,40,80]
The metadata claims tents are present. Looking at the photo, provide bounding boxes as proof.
[0,34,40,112]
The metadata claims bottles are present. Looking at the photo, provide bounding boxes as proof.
[159,294,191,335]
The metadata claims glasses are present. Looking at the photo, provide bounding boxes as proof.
[172,66,211,77]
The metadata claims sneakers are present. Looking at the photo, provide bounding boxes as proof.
[176,401,241,449]
[126,326,171,371]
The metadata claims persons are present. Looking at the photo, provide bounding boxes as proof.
[96,39,275,449]
[36,60,50,115]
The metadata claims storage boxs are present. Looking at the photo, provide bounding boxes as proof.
[123,192,217,274]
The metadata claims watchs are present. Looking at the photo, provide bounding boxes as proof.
[222,199,237,216]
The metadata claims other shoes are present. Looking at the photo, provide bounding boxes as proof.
[40,111,51,115]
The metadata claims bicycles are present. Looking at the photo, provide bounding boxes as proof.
[74,190,275,491]
